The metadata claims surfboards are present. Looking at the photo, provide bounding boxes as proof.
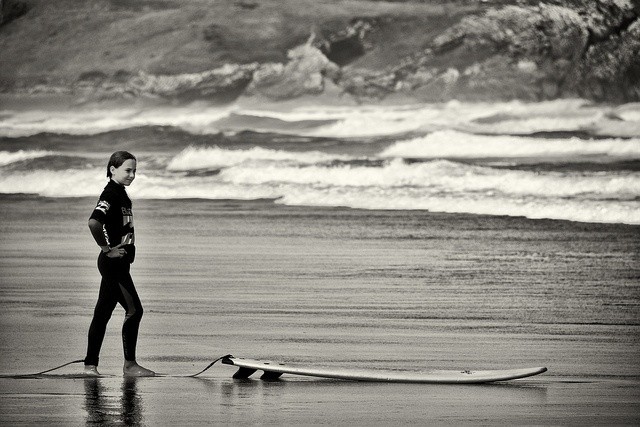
[221,353,547,383]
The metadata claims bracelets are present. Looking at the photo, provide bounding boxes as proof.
[103,249,111,254]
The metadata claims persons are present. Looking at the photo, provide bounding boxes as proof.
[81,150,156,378]
